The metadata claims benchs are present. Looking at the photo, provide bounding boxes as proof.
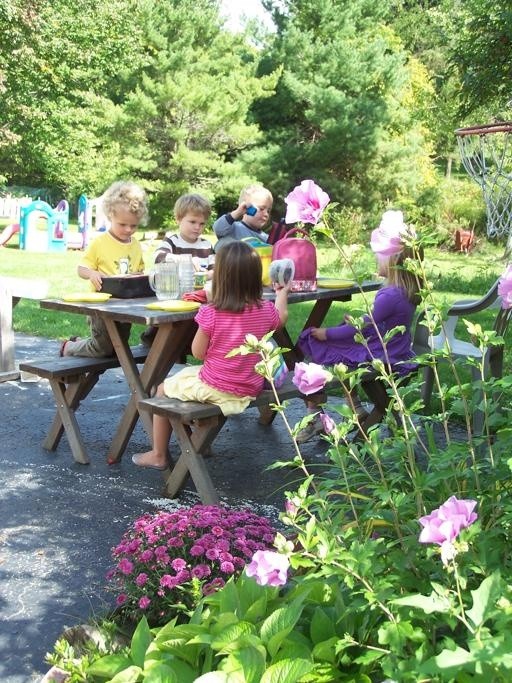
[19,343,271,464]
[138,365,427,505]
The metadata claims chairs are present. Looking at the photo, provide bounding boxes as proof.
[412,277,512,436]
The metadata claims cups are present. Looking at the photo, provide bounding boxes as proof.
[167,254,194,296]
[150,261,180,300]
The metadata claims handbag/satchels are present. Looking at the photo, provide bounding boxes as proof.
[270,227,318,293]
[240,236,273,287]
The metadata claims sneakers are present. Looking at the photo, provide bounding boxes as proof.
[131,451,169,471]
[295,395,371,444]
[346,410,369,435]
[290,415,329,445]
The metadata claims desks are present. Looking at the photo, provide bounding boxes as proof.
[41,281,382,463]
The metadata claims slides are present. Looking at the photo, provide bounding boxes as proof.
[0,224,20,245]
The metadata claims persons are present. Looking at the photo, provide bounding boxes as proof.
[293,236,424,445]
[212,181,276,252]
[130,239,296,471]
[138,191,217,357]
[53,178,155,373]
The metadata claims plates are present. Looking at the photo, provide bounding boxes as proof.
[317,279,356,290]
[62,294,112,305]
[142,300,202,312]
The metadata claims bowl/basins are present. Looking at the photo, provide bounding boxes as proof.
[268,259,295,289]
[95,274,157,300]
